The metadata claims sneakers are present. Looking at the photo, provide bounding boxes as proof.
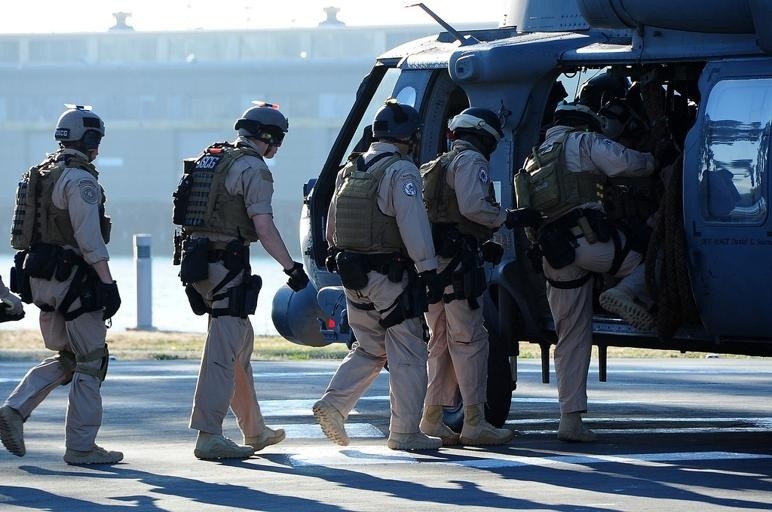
[387,428,443,452]
[193,431,254,459]
[0,404,29,458]
[311,398,352,447]
[62,445,124,467]
[241,426,286,452]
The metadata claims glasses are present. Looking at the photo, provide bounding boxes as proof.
[605,111,628,124]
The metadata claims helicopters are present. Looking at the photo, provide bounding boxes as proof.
[270,1,772,441]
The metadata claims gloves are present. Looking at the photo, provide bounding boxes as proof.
[2,294,23,317]
[100,280,122,322]
[507,207,543,230]
[282,261,311,292]
[419,268,447,306]
[658,139,679,165]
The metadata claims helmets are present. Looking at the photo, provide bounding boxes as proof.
[54,109,106,148]
[453,107,504,151]
[233,106,288,137]
[555,99,604,134]
[371,102,420,141]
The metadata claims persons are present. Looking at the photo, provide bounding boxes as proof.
[170,100,311,462]
[418,103,545,448]
[0,106,125,467]
[312,94,445,452]
[511,103,671,442]
[1,276,28,325]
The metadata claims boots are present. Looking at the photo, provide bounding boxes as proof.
[558,414,599,445]
[417,402,460,446]
[598,285,657,331]
[459,403,514,446]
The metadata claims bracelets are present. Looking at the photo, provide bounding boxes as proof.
[282,262,298,274]
